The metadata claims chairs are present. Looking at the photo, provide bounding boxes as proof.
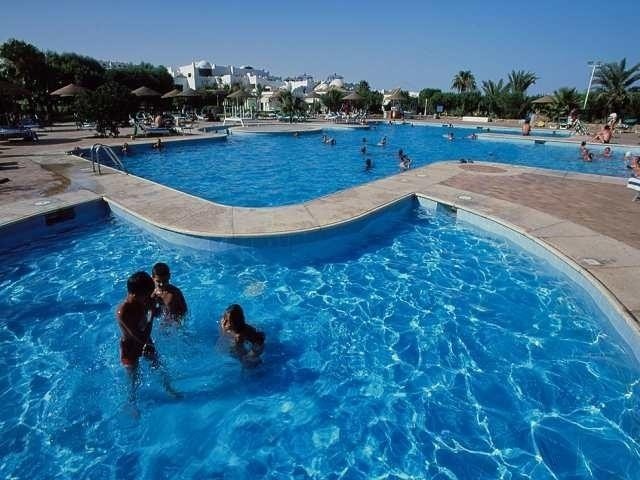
[627,176,640,201]
[0,118,42,143]
[139,123,171,136]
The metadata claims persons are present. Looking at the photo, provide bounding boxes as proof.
[521,118,532,136]
[580,140,640,179]
[448,131,455,140]
[153,137,164,148]
[150,262,189,330]
[121,143,128,153]
[590,119,618,144]
[217,303,267,372]
[115,270,185,418]
[322,134,413,173]
[154,113,167,127]
[24,128,40,144]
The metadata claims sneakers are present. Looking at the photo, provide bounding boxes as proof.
[457,158,473,163]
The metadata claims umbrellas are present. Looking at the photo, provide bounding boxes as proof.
[60,93,83,97]
[129,87,163,97]
[531,96,553,104]
[304,91,321,98]
[226,88,257,98]
[160,88,182,99]
[176,87,202,97]
[385,93,407,101]
[48,82,83,94]
[342,91,367,100]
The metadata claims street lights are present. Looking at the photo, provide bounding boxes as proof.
[583,62,602,108]
